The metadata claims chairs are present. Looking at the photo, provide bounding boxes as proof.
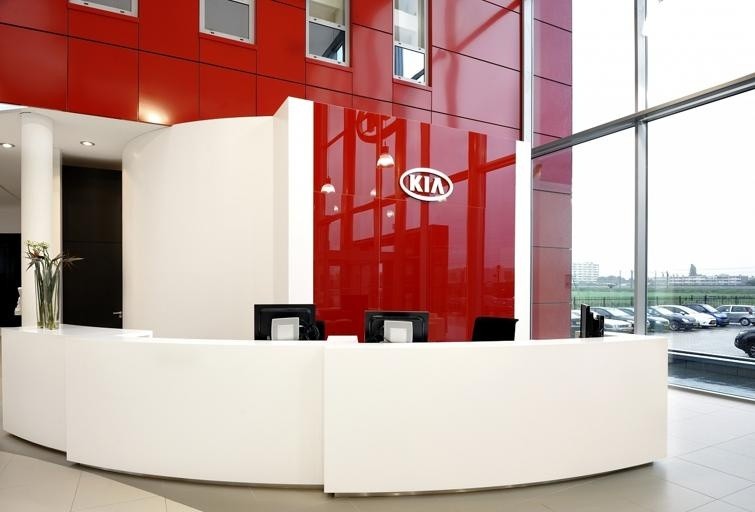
[472,316,518,341]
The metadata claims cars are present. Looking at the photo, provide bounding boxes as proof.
[715,304,755,326]
[734,329,755,358]
[590,307,650,331]
[621,306,670,332]
[572,309,633,334]
[315,280,515,335]
[653,305,698,331]
[657,304,716,329]
[571,312,581,335]
[687,302,729,329]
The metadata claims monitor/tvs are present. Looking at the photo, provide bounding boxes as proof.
[364,310,429,343]
[254,304,315,340]
[580,303,604,338]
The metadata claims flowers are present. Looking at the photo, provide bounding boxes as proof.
[24,239,85,326]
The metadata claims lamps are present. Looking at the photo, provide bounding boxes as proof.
[321,105,335,194]
[377,116,395,168]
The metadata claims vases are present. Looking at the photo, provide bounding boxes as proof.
[34,268,62,329]
